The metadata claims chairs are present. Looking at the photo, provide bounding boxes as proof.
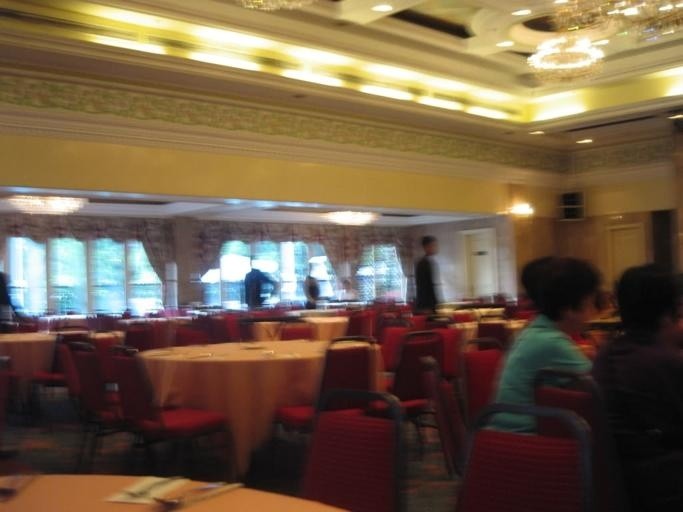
[460,349,501,411]
[101,345,231,476]
[383,331,452,479]
[281,325,317,340]
[32,333,89,387]
[455,403,595,512]
[476,322,503,348]
[301,387,409,512]
[62,334,101,473]
[345,310,373,338]
[268,336,374,468]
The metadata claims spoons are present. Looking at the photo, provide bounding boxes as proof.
[155,479,227,506]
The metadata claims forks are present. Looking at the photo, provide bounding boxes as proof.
[123,475,186,496]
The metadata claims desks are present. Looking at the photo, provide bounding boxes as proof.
[136,339,382,472]
[0,333,57,374]
[0,473,347,512]
[254,316,348,339]
[452,319,527,348]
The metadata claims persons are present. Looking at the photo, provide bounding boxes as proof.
[479,257,602,439]
[592,263,683,512]
[215,231,444,321]
